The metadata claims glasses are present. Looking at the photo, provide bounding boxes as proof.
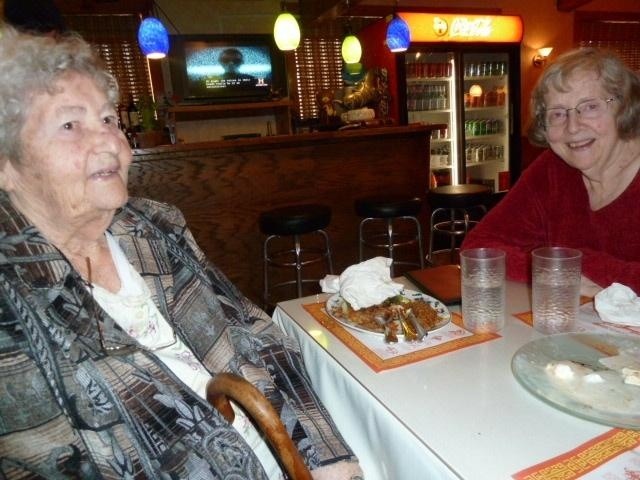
[537,98,616,126]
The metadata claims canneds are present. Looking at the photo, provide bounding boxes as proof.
[464,61,507,164]
[403,60,452,168]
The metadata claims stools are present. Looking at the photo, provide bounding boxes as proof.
[259,203,334,315]
[354,193,425,275]
[426,182,492,266]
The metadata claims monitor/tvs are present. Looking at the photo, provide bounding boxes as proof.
[168,34,286,105]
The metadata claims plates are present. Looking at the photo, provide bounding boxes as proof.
[511,330,640,433]
[324,289,451,336]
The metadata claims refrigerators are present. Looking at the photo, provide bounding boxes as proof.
[350,10,525,196]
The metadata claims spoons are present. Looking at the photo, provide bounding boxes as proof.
[373,306,399,345]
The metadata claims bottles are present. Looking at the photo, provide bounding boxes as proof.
[119,92,178,147]
[406,59,507,184]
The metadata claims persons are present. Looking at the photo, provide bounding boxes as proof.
[0,21,365,479]
[457,46,639,297]
[203,48,260,89]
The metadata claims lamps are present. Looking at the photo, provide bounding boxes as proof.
[137,16,172,59]
[273,13,301,51]
[342,35,362,64]
[533,46,554,66]
[386,18,411,52]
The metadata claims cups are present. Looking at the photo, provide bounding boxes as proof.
[530,245,583,336]
[459,247,507,337]
